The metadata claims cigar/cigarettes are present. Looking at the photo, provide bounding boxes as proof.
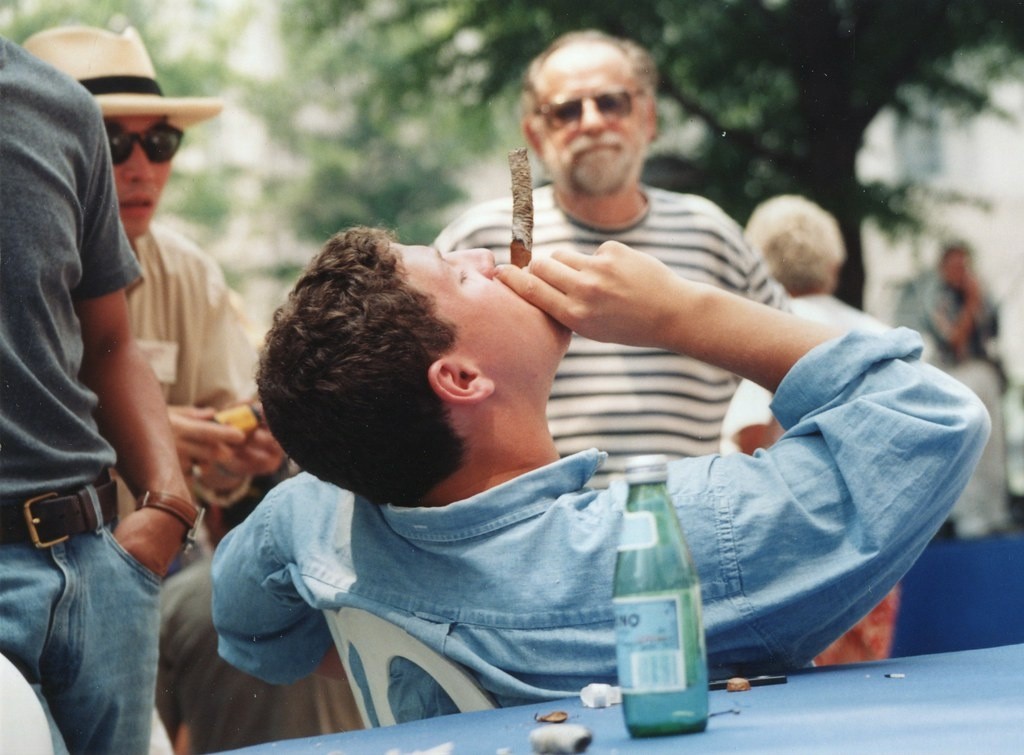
[505,144,536,268]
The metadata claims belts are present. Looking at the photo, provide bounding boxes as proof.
[0,478,119,548]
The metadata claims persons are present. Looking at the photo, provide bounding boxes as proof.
[714,194,901,669]
[204,226,995,725]
[18,25,331,755]
[427,27,785,495]
[0,32,200,755]
[910,236,1015,537]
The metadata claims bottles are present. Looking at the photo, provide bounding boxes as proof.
[610,454,709,740]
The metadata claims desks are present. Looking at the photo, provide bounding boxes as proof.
[206,638,1024,755]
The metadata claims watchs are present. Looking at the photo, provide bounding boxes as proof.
[134,490,205,550]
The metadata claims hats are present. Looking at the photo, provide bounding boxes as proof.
[22,27,223,129]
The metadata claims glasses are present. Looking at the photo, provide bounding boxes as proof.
[533,90,644,130]
[102,120,185,167]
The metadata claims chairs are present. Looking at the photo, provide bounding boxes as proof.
[322,604,501,733]
